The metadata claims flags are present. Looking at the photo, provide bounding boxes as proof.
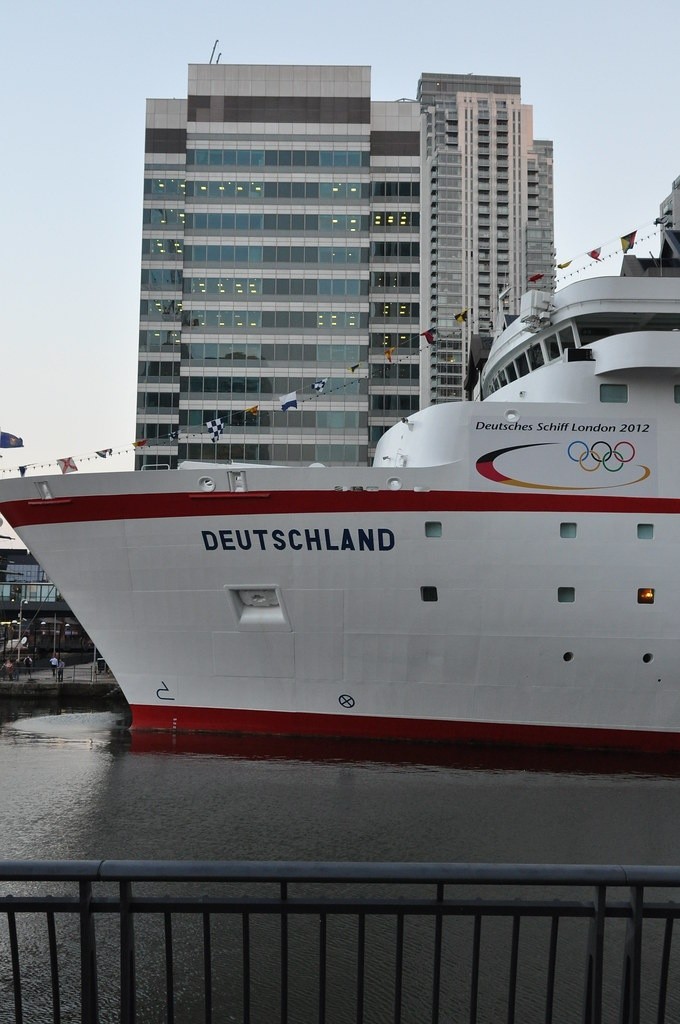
[278,390,297,412]
[619,229,638,254]
[56,456,79,476]
[0,431,24,449]
[206,417,226,444]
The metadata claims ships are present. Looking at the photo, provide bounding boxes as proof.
[0,274,679,766]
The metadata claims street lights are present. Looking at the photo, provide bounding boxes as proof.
[18,599,28,661]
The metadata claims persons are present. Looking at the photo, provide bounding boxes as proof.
[5,659,14,682]
[49,656,58,677]
[57,658,65,682]
[23,655,32,676]
